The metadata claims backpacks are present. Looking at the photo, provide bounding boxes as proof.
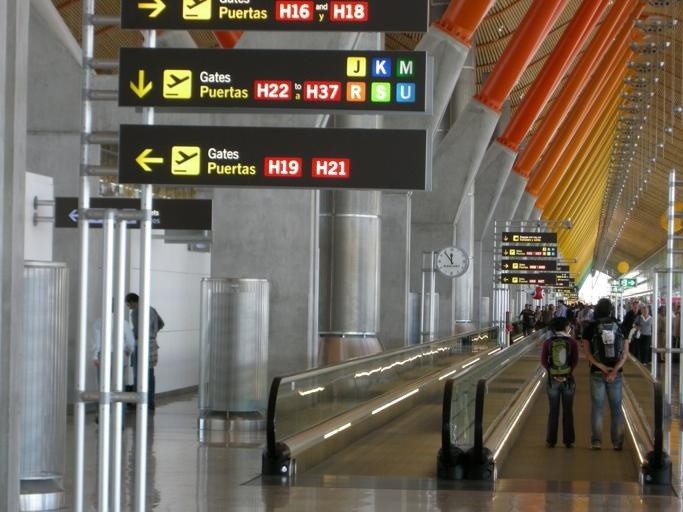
[547,336,573,376]
[593,320,624,367]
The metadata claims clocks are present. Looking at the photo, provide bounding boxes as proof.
[435,244,469,278]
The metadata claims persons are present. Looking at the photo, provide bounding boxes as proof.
[580,300,629,452]
[123,290,165,412]
[538,315,579,449]
[516,299,680,367]
[90,295,136,430]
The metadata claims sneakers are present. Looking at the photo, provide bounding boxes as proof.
[544,441,623,450]
